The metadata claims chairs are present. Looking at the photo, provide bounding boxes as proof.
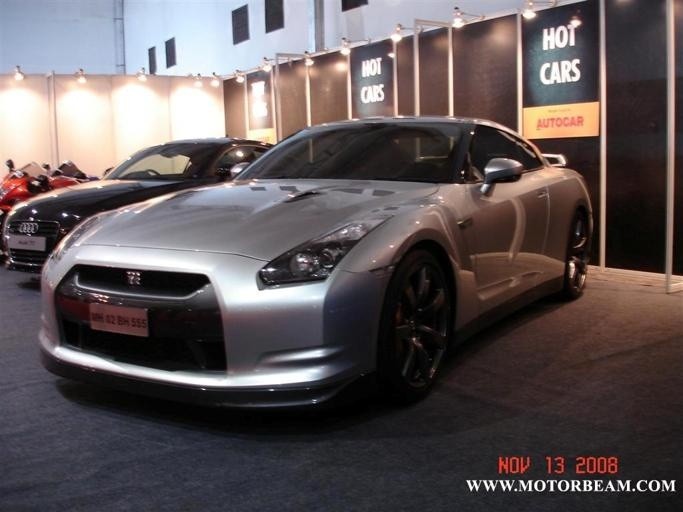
[344,134,488,182]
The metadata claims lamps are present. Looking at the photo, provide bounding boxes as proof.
[73,68,87,84]
[135,67,148,83]
[12,66,25,82]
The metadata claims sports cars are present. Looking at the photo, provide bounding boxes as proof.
[0,112,596,415]
